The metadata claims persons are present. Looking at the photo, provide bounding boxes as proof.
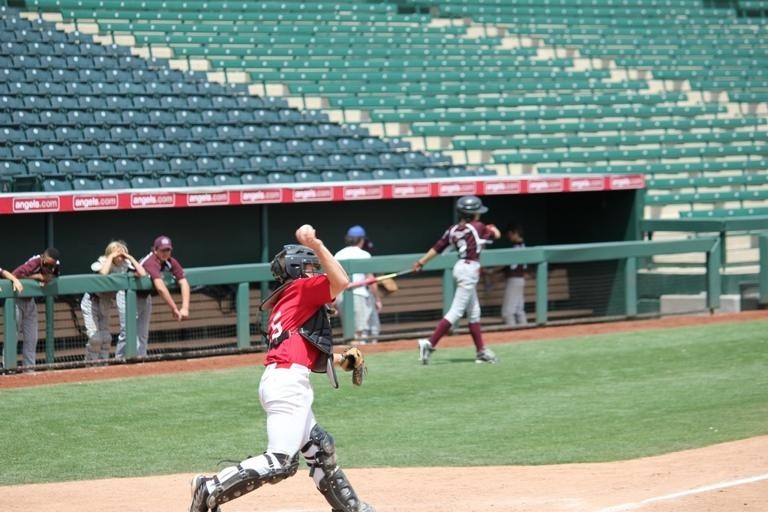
[361,238,383,345]
[188,223,376,511]
[332,224,372,344]
[492,218,529,326]
[0,266,25,295]
[409,195,502,366]
[115,234,192,363]
[77,238,148,369]
[1,246,66,373]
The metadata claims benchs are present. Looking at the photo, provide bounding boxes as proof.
[0,268,594,371]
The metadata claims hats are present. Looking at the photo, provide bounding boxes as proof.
[347,225,366,237]
[155,236,172,250]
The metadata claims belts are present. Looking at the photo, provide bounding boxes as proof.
[276,362,292,368]
[464,259,470,264]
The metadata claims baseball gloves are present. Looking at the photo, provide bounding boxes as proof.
[339,348,365,385]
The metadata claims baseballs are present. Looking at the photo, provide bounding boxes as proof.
[298,225,315,235]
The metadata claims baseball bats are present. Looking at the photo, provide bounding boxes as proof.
[343,267,421,290]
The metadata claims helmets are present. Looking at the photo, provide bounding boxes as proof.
[458,196,488,214]
[270,244,320,283]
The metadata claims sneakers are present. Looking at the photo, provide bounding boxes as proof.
[475,350,497,364]
[418,338,435,364]
[188,474,210,512]
